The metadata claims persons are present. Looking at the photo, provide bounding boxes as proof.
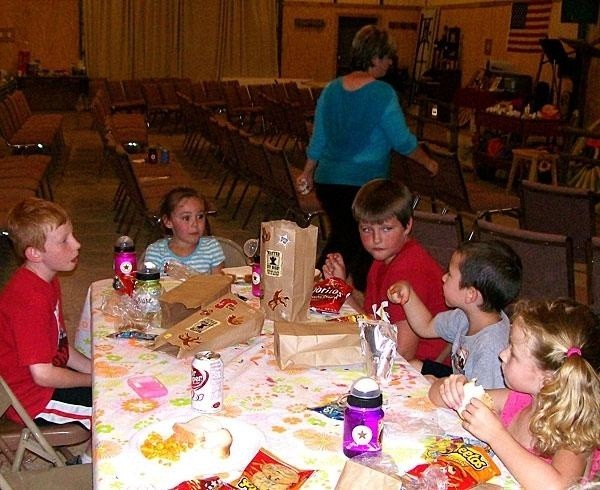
[297,24,440,294]
[386,238,524,391]
[0,195,93,468]
[427,297,599,490]
[143,187,226,285]
[321,177,455,364]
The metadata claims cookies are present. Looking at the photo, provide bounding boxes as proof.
[251,463,299,490]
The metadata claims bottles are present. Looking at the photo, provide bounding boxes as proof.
[343,376,384,459]
[244,239,262,298]
[488,102,536,118]
[110,237,136,295]
[144,144,170,164]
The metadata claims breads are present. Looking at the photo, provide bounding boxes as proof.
[456,378,495,421]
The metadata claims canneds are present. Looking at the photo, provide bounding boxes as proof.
[148,148,158,164]
[191,350,224,414]
[160,149,169,164]
